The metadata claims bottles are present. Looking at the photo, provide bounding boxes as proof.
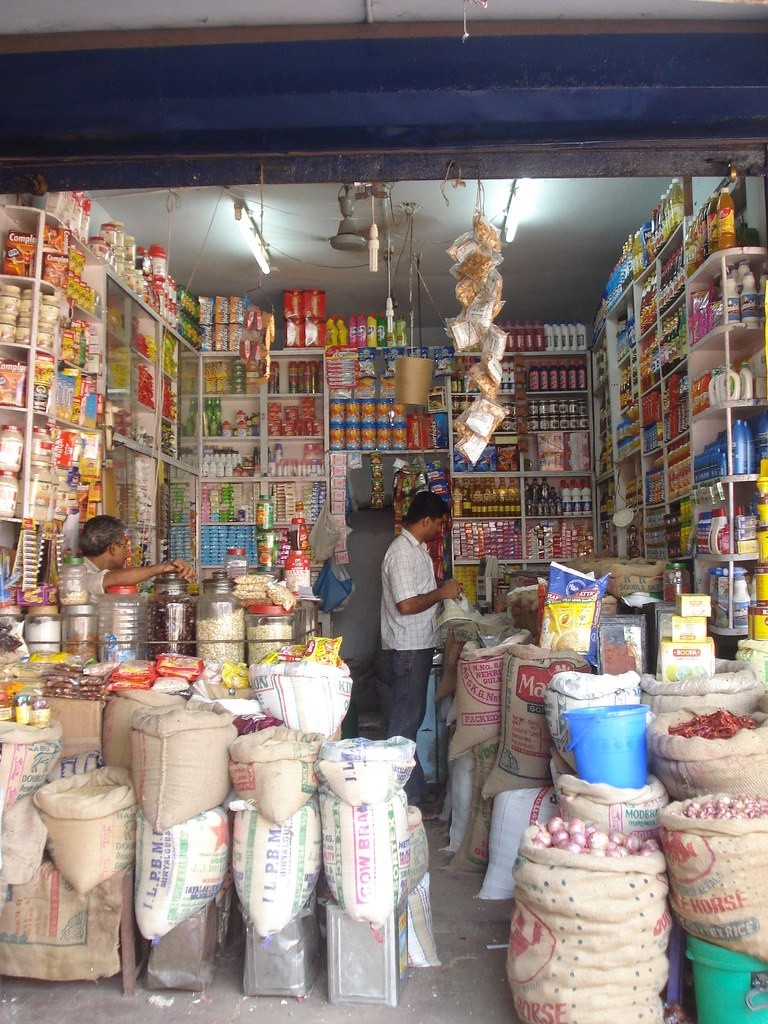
[57,558,87,604]
[663,563,691,605]
[609,178,768,343]
[184,398,260,436]
[453,478,592,515]
[178,444,324,476]
[326,313,408,347]
[267,360,324,393]
[224,549,248,580]
[450,356,586,393]
[497,320,587,352]
[0,570,316,668]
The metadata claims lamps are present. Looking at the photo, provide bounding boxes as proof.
[395,217,434,405]
[505,178,529,243]
[233,203,271,275]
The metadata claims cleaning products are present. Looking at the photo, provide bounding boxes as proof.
[639,359,697,563]
[708,566,756,629]
[693,408,768,484]
[713,260,768,323]
[498,320,587,393]
[326,312,408,347]
[696,490,758,555]
[561,480,592,515]
[691,359,767,416]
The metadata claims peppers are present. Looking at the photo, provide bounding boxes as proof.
[667,706,759,741]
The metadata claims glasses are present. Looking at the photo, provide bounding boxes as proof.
[107,541,129,551]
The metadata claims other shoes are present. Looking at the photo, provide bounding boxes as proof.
[417,803,440,821]
[421,791,437,802]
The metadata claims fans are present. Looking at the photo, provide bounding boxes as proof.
[271,184,437,257]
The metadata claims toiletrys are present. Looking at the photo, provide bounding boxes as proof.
[200,441,326,478]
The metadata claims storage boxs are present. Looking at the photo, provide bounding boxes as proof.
[302,289,326,318]
[284,317,305,347]
[495,436,518,470]
[671,615,707,642]
[564,433,589,471]
[475,445,496,471]
[675,593,712,617]
[660,636,715,681]
[452,445,474,472]
[283,290,304,318]
[305,317,326,347]
[406,413,433,450]
[538,432,564,471]
[430,413,449,448]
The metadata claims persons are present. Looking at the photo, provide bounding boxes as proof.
[79,515,197,603]
[378,490,462,820]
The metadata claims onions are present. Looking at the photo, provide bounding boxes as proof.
[674,795,768,820]
[530,815,659,856]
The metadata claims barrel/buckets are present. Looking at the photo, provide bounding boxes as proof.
[393,357,436,406]
[685,933,768,1024]
[562,704,651,789]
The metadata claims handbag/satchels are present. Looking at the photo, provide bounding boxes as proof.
[313,560,352,613]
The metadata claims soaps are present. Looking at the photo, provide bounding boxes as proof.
[452,521,522,559]
[525,520,593,559]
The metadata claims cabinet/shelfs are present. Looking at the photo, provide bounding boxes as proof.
[0,173,768,635]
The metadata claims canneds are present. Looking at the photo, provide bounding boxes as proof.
[527,399,589,431]
